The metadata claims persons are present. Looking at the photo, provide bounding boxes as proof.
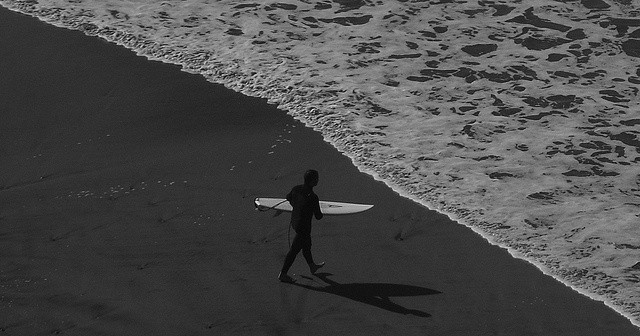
[278,169,325,283]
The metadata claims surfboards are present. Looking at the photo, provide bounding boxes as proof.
[255,198,375,215]
